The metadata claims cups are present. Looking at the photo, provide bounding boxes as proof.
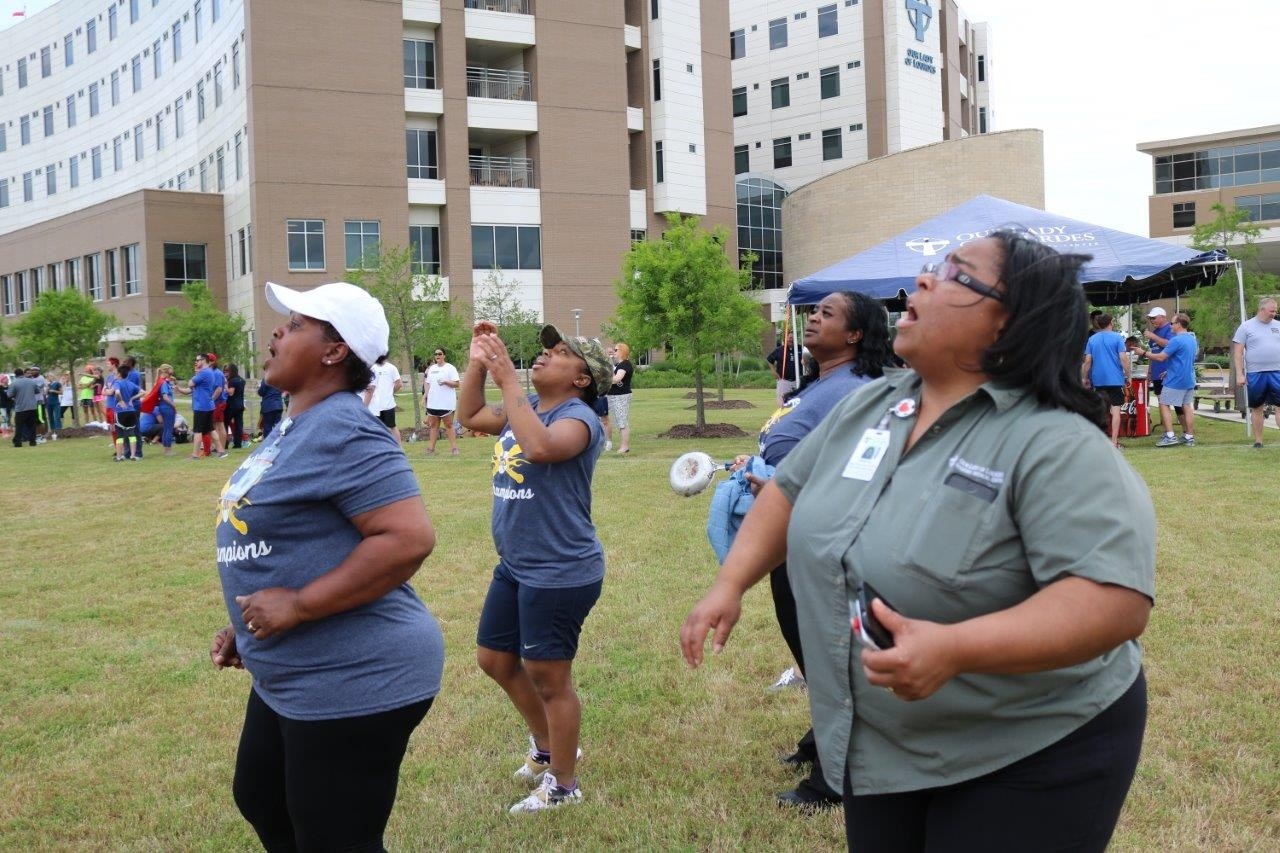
[52,434,57,441]
[1137,364,1149,375]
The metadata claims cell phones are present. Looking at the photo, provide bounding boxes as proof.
[857,580,896,653]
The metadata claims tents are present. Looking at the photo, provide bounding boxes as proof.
[788,193,1254,441]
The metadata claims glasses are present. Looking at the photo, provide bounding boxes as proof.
[1171,322,1178,325]
[434,352,442,356]
[1147,317,1156,320]
[197,359,204,362]
[920,260,1008,304]
[614,348,622,352]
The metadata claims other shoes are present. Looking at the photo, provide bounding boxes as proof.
[131,456,144,462]
[1116,442,1126,450]
[779,764,842,816]
[1155,435,1178,447]
[1183,436,1196,447]
[450,447,460,455]
[217,451,229,459]
[149,440,161,446]
[1252,442,1264,449]
[781,727,819,767]
[605,441,613,451]
[424,448,435,454]
[113,454,127,462]
[617,447,629,454]
[186,454,200,461]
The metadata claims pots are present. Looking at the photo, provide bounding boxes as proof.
[668,452,737,496]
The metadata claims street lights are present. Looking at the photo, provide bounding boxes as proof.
[570,308,584,337]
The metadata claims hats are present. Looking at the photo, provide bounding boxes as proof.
[0,374,9,383]
[1146,306,1166,319]
[540,323,614,396]
[65,371,70,374]
[85,364,95,371]
[31,365,40,371]
[95,365,103,372]
[264,281,390,369]
[208,353,216,362]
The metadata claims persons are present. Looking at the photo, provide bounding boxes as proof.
[206,281,443,853]
[680,228,1280,853]
[0,321,632,811]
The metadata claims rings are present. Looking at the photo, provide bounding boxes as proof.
[885,685,894,695]
[247,621,257,632]
[490,354,497,360]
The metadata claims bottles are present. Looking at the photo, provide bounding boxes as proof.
[1120,383,1137,437]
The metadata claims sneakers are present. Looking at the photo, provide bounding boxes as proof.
[767,667,806,694]
[509,772,583,815]
[513,734,581,786]
